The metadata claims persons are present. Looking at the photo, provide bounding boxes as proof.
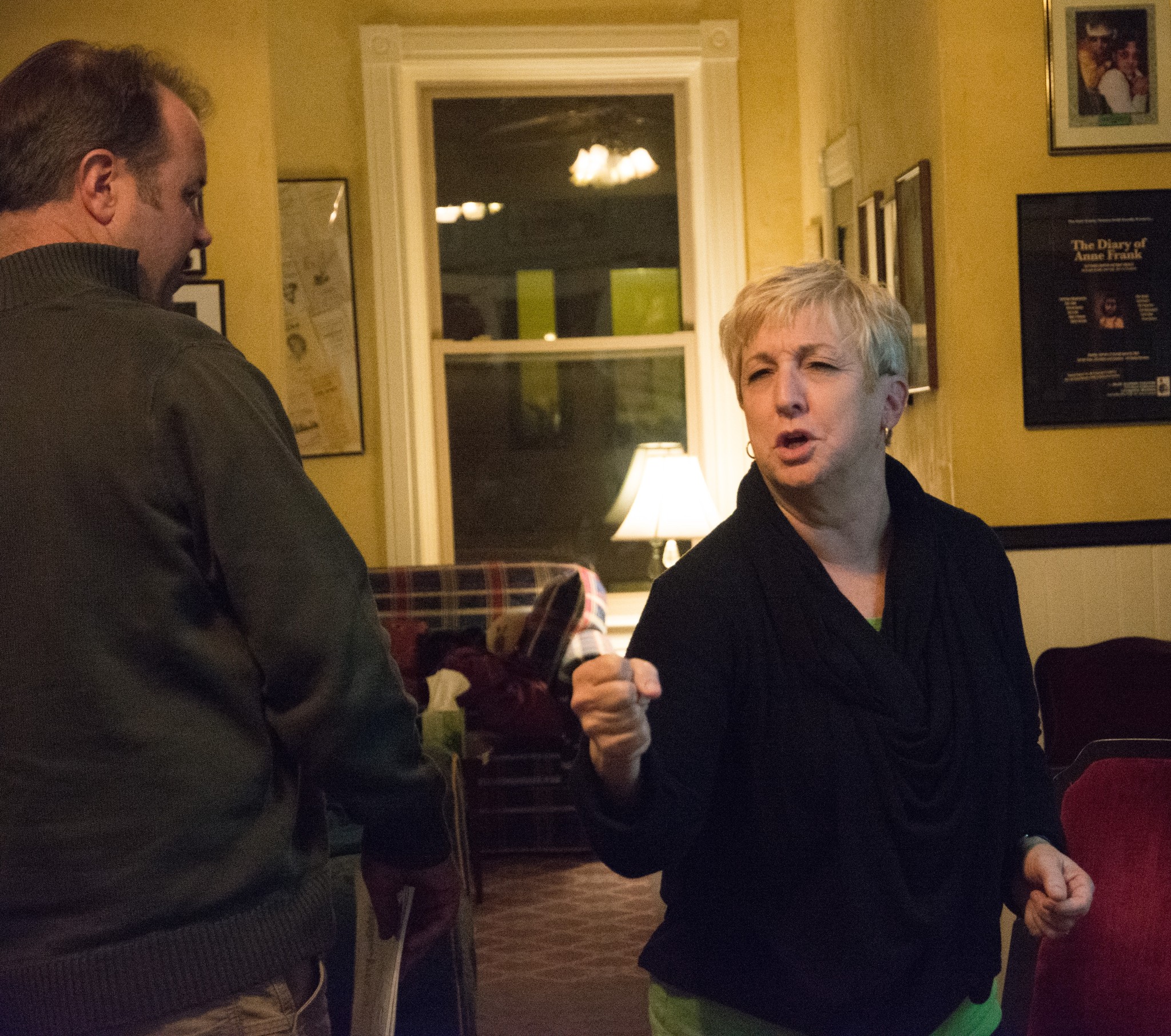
[571,263,1095,1035]
[1075,13,1149,113]
[0,41,470,1036]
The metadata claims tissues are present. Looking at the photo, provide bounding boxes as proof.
[420,667,473,758]
